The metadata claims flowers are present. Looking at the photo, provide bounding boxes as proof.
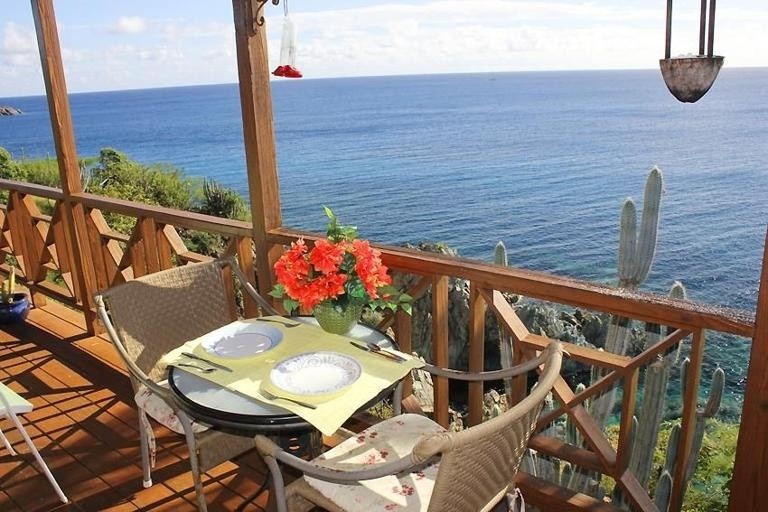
[267,203,416,318]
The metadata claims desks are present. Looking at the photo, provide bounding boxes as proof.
[168,313,402,512]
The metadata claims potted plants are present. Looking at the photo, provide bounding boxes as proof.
[0,262,30,326]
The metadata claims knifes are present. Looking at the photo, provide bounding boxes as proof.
[183,349,236,371]
[350,340,400,364]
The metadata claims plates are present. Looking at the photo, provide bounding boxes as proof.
[201,322,282,361]
[270,351,362,394]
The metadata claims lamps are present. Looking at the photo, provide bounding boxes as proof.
[657,0,728,105]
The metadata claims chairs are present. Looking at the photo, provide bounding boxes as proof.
[93,256,281,512]
[253,342,563,512]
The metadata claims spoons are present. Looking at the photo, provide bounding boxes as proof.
[179,363,218,374]
[367,340,407,362]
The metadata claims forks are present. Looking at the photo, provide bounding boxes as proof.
[259,390,317,412]
[256,315,303,331]
